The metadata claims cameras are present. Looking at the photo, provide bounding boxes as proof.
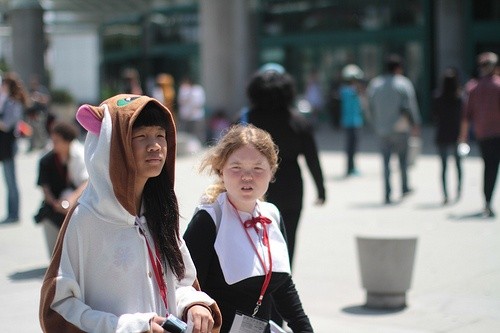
[160,313,188,333]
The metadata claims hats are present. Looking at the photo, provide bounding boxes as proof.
[342,64,364,80]
[476,52,498,77]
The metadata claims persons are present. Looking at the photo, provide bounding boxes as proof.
[39,93,222,333]
[34,120,90,261]
[243,69,325,273]
[0,51,500,224]
[183,120,313,332]
